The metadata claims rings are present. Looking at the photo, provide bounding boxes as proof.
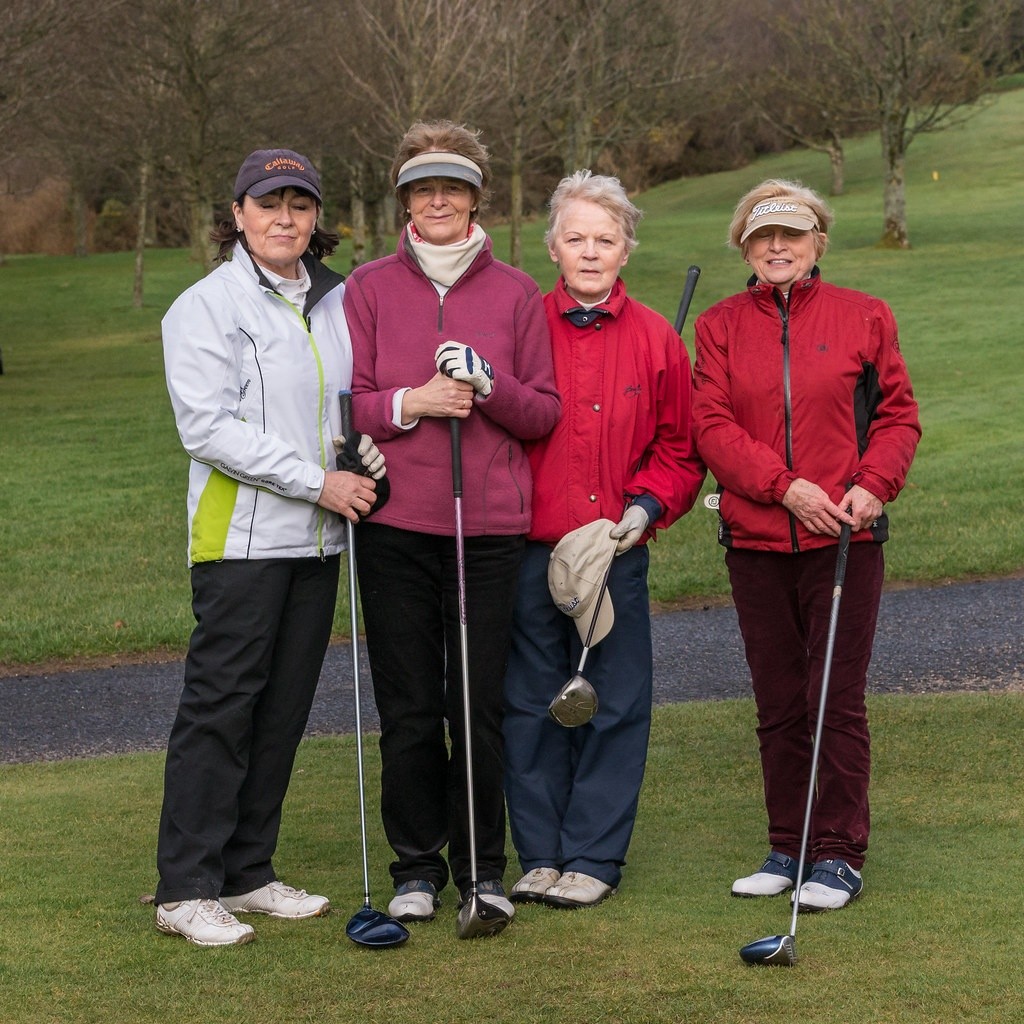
[461,400,465,408]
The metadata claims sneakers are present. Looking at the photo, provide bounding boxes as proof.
[544,871,618,907]
[790,860,863,913]
[732,848,809,898]
[218,880,330,919]
[155,899,256,946]
[511,866,561,901]
[465,880,515,921]
[388,879,439,920]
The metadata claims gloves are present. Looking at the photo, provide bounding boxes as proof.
[434,340,494,396]
[608,503,648,556]
[332,433,387,480]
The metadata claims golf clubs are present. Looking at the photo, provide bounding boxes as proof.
[546,266,703,729]
[737,483,854,966]
[453,412,517,941]
[340,394,410,949]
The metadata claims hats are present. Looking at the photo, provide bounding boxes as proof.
[547,519,619,649]
[394,153,483,190]
[233,149,322,206]
[740,196,820,244]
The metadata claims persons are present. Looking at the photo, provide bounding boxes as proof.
[155,149,387,948]
[342,119,562,921]
[690,179,922,911]
[503,169,707,910]
